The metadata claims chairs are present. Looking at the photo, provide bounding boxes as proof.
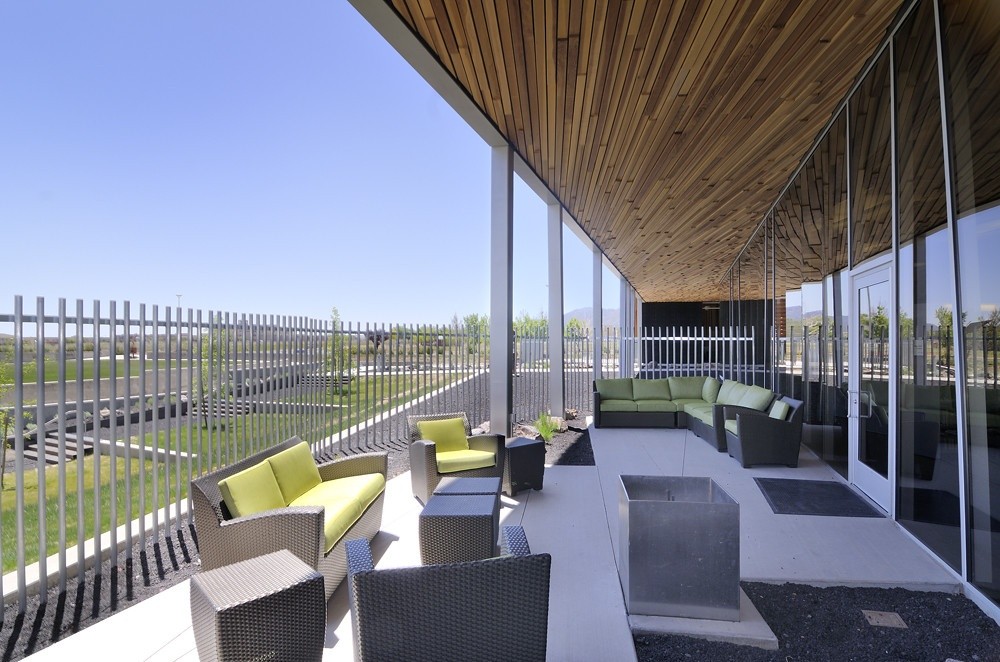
[344,526,552,662]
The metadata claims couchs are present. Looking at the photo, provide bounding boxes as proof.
[407,411,505,505]
[191,434,389,604]
[593,375,804,470]
[810,379,999,480]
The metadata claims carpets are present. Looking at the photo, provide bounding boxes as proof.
[753,476,885,519]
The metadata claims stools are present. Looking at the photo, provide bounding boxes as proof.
[190,549,327,662]
[420,475,501,565]
[501,436,546,496]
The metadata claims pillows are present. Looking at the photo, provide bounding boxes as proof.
[769,400,789,419]
[702,376,720,404]
[417,418,469,453]
[218,441,322,521]
